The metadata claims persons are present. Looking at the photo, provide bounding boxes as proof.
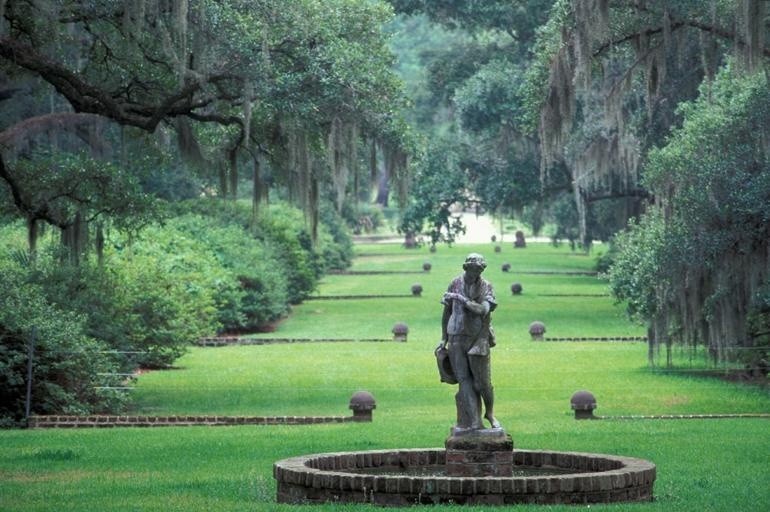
[440,252,498,429]
